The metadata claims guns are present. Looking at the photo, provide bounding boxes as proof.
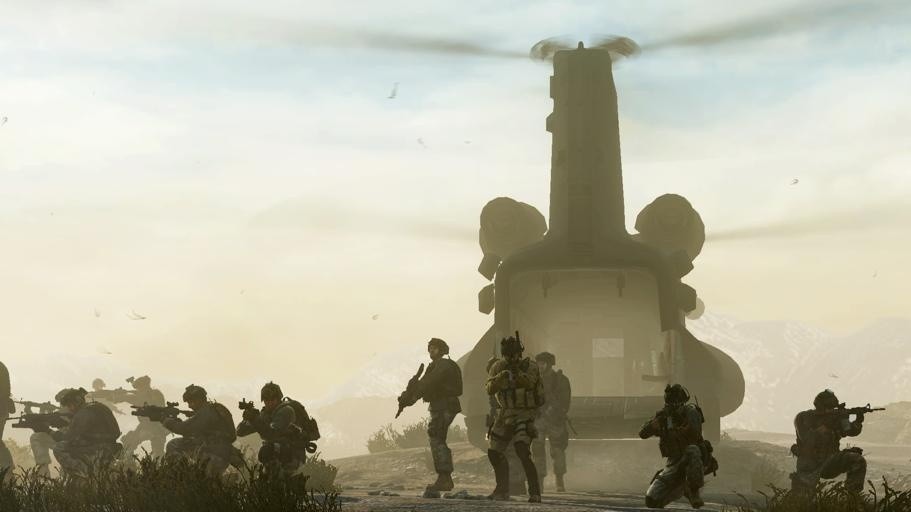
[395,364,424,418]
[809,403,885,420]
[5,401,72,429]
[85,387,136,405]
[239,401,253,410]
[130,403,194,421]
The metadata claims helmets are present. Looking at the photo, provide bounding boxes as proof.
[535,350,556,366]
[427,337,450,355]
[53,375,150,411]
[499,336,523,354]
[182,383,207,401]
[813,390,839,408]
[260,381,284,401]
[662,383,689,404]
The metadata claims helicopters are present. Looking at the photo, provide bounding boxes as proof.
[244,2,904,454]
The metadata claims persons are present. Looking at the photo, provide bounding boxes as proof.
[395,336,463,491]
[147,382,235,475]
[788,390,867,509]
[528,351,571,493]
[237,379,320,479]
[0,361,126,512]
[638,382,719,510]
[486,334,543,504]
[485,356,526,496]
[113,375,170,472]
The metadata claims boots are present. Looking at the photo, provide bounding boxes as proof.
[428,472,455,492]
[486,481,509,500]
[538,475,565,493]
[526,483,542,503]
[682,480,706,509]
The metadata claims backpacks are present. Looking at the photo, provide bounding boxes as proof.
[270,397,322,441]
[211,400,237,444]
[81,399,122,441]
